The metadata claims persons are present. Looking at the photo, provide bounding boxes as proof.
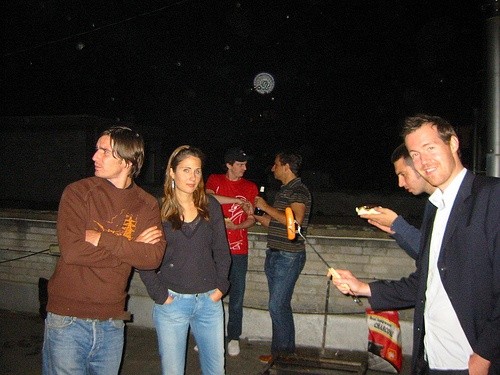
[41,127,166,375]
[245,152,311,364]
[328,112,500,375]
[205,146,259,357]
[137,145,232,375]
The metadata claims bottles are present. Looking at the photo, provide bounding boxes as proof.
[254,185,265,216]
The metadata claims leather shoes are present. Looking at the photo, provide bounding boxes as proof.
[259,354,272,364]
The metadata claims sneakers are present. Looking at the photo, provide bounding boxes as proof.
[228,340,240,357]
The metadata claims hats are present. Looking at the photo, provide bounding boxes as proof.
[223,145,250,161]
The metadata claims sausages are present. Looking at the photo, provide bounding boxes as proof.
[285,207,296,240]
